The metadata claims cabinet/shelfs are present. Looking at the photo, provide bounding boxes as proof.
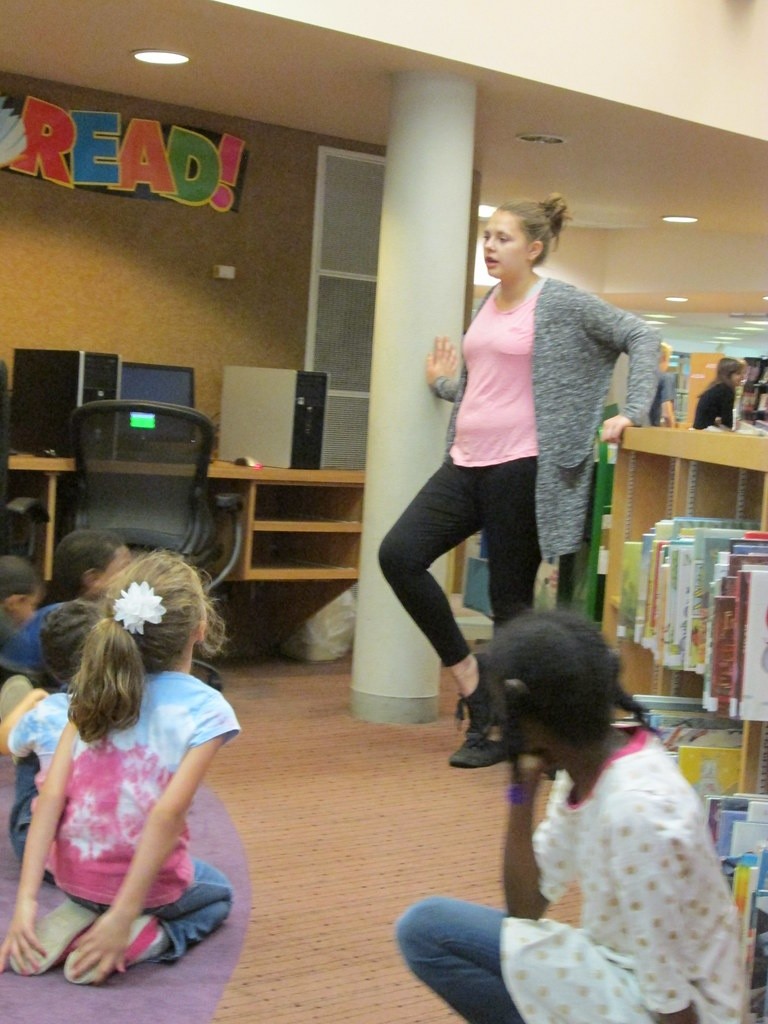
[587,421,768,793]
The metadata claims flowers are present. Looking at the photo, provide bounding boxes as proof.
[112,581,167,635]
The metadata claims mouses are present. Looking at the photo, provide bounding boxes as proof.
[235,456,260,467]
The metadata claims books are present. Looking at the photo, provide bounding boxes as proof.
[464,506,767,1023]
[669,354,768,435]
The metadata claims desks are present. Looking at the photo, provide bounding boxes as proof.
[10,451,366,584]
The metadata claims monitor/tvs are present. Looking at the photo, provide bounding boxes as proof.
[121,361,194,444]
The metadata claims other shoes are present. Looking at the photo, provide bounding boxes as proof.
[9,901,98,974]
[64,915,161,984]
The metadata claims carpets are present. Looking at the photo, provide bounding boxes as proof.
[1,749,253,1023]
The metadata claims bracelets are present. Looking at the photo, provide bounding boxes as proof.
[507,785,533,806]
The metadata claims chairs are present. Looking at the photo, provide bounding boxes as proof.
[52,397,248,690]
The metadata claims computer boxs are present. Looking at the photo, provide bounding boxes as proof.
[219,365,327,468]
[10,349,121,459]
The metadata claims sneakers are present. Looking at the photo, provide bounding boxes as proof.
[456,653,494,742]
[449,736,511,764]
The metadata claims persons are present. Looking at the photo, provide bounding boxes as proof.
[0,530,241,987]
[694,357,746,431]
[649,344,677,427]
[392,606,752,1024]
[380,191,663,770]
